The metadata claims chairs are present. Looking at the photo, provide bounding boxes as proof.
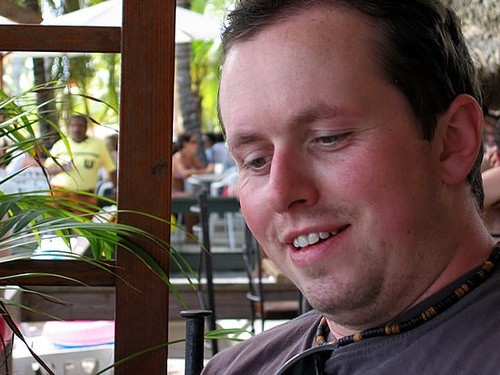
[208,171,247,249]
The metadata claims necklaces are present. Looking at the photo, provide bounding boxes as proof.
[315,241,500,347]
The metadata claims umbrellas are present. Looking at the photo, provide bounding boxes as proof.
[6,0,227,57]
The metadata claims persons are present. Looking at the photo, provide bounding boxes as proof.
[172,0,500,375]
[0,114,120,258]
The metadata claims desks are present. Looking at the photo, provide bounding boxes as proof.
[185,174,213,194]
